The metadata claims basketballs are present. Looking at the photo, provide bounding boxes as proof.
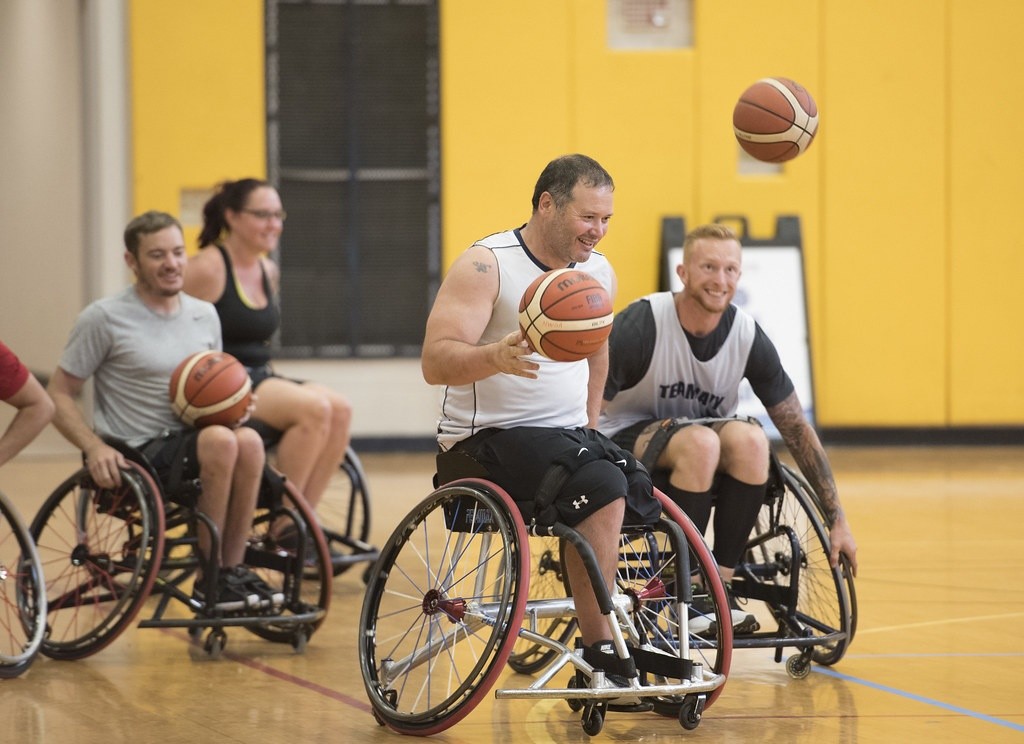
[170,351,254,431]
[518,268,615,362]
[732,78,819,164]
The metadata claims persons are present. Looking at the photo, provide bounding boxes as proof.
[180,178,352,567]
[47,211,290,611]
[0,339,55,464]
[420,155,663,706]
[595,223,859,638]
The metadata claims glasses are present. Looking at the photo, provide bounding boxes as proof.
[243,208,288,221]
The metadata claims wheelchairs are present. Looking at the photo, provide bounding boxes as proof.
[361,472,736,738]
[612,451,858,717]
[0,445,335,677]
[183,409,380,578]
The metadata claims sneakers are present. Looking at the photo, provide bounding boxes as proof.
[574,639,645,705]
[676,583,760,638]
[263,523,340,567]
[191,568,283,614]
